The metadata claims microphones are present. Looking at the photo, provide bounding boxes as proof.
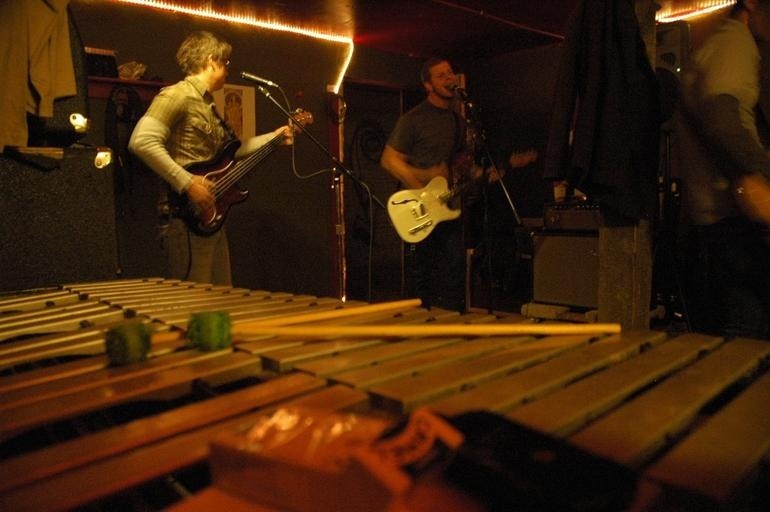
[442,80,474,109]
[238,66,278,94]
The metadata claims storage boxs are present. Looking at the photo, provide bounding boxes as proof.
[87,75,174,101]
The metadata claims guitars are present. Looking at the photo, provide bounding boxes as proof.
[168,109,315,233]
[388,150,538,243]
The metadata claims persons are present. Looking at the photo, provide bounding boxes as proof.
[127,29,297,288]
[657,0,770,337]
[381,53,507,314]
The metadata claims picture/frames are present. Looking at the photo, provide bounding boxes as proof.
[214,83,257,145]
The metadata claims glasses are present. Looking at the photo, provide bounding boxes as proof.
[221,57,232,67]
